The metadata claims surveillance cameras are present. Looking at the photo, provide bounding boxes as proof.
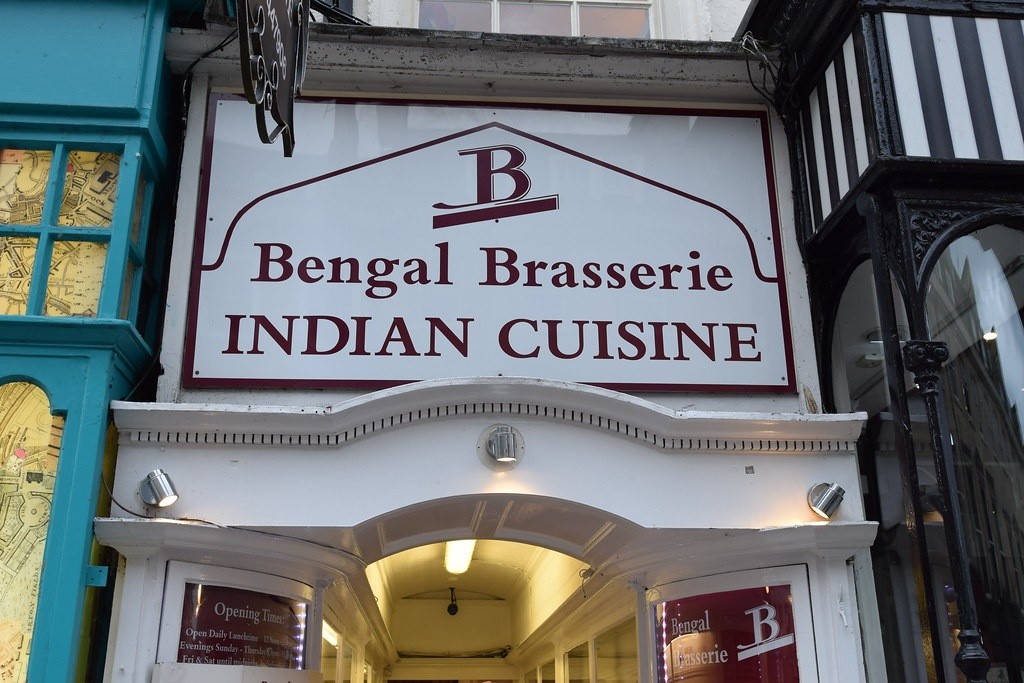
[448,604,458,615]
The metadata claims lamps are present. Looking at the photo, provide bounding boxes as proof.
[486,426,516,462]
[807,482,845,519]
[140,469,179,508]
[443,538,475,575]
